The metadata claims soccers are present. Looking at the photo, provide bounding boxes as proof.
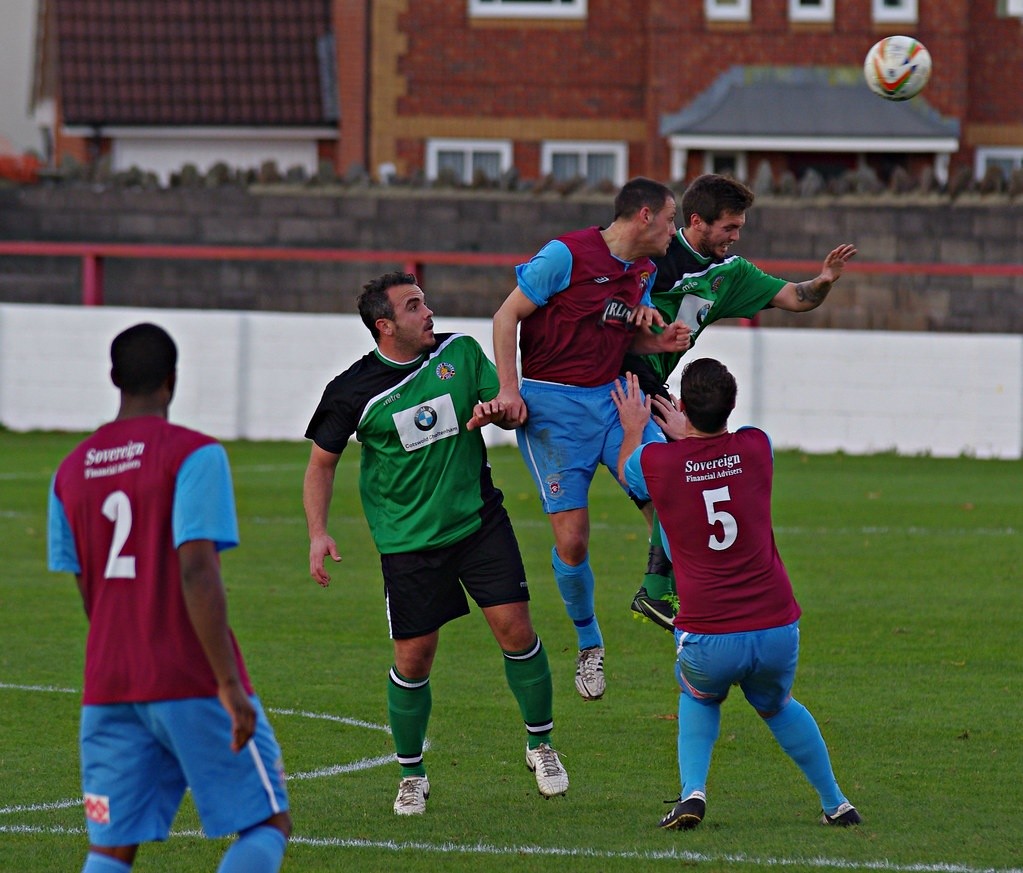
[862,35,932,101]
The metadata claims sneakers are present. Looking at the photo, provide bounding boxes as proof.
[575,647,606,700]
[394,774,430,815]
[815,802,861,827]
[630,586,677,634]
[659,791,707,829]
[526,741,569,798]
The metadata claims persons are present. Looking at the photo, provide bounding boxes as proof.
[611,357,857,828]
[48,323,292,873]
[301,273,569,818]
[493,177,690,696]
[623,170,859,632]
